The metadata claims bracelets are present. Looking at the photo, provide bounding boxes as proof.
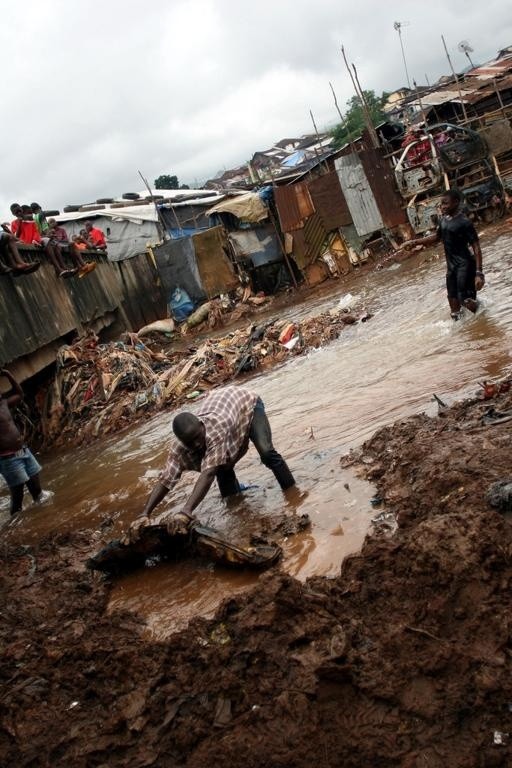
[411,239,418,244]
[474,270,483,278]
[180,507,195,518]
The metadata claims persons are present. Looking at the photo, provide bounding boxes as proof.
[0,203,106,279]
[404,189,485,322]
[129,386,295,545]
[400,127,452,166]
[0,368,44,515]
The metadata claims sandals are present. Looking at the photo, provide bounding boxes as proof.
[0,258,95,278]
